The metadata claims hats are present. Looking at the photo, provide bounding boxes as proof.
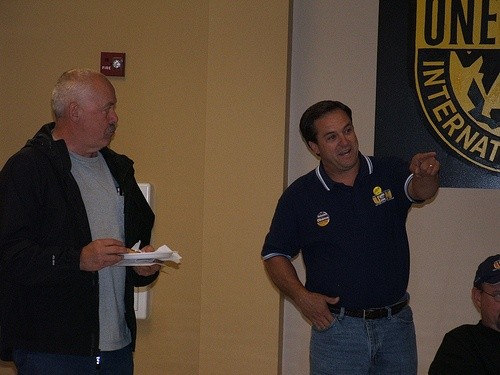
[472,254,500,286]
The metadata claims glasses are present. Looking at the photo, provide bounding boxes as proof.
[476,287,500,302]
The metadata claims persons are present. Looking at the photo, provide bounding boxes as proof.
[0,67,160,374]
[427,254,500,375]
[259,99,439,373]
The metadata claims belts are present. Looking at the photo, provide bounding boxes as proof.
[329,300,408,319]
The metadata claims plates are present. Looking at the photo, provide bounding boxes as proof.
[117,252,172,265]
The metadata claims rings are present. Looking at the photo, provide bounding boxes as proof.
[428,162,435,169]
